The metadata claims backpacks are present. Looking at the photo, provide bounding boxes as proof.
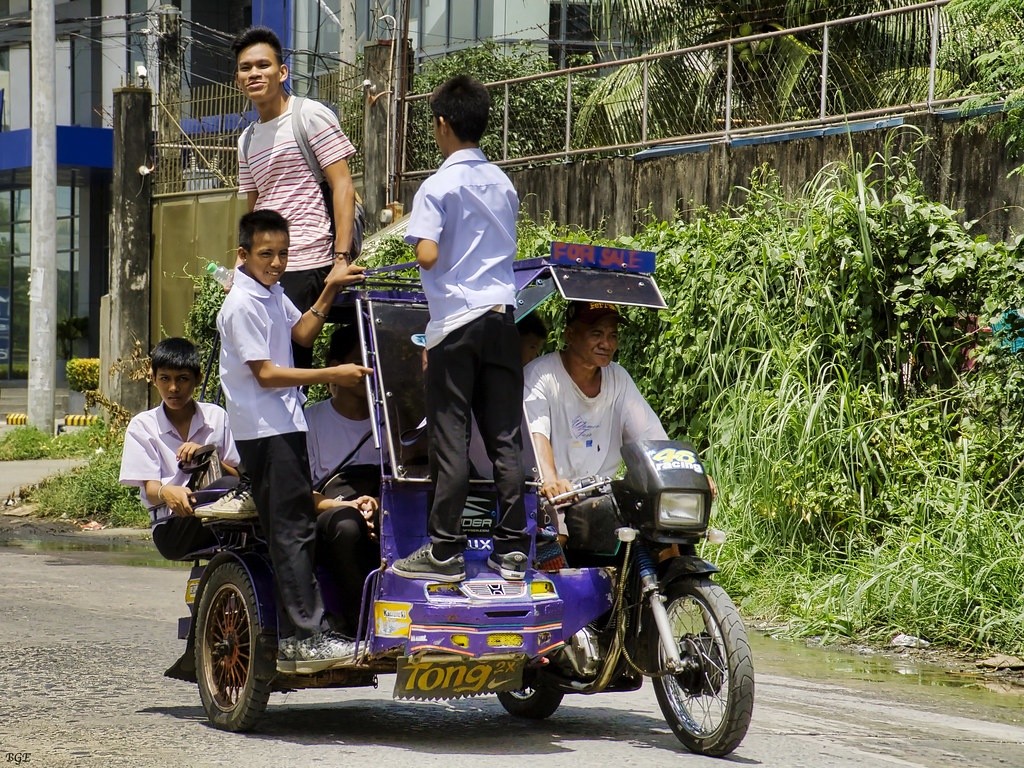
[244,96,363,259]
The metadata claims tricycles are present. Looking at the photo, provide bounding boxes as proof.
[163,240,757,759]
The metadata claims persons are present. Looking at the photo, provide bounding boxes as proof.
[411,299,717,573]
[303,74,533,644]
[195,26,365,520]
[118,338,267,561]
[215,210,373,673]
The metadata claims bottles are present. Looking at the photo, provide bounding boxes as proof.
[207,263,234,290]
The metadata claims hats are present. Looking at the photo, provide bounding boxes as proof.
[564,301,631,326]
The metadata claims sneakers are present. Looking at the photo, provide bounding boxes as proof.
[296,631,371,673]
[392,543,465,583]
[490,548,528,580]
[195,482,246,516]
[215,489,258,517]
[276,634,299,673]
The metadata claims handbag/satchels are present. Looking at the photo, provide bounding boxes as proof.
[325,462,391,510]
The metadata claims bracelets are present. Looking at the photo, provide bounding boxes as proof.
[310,306,328,318]
[157,484,165,504]
